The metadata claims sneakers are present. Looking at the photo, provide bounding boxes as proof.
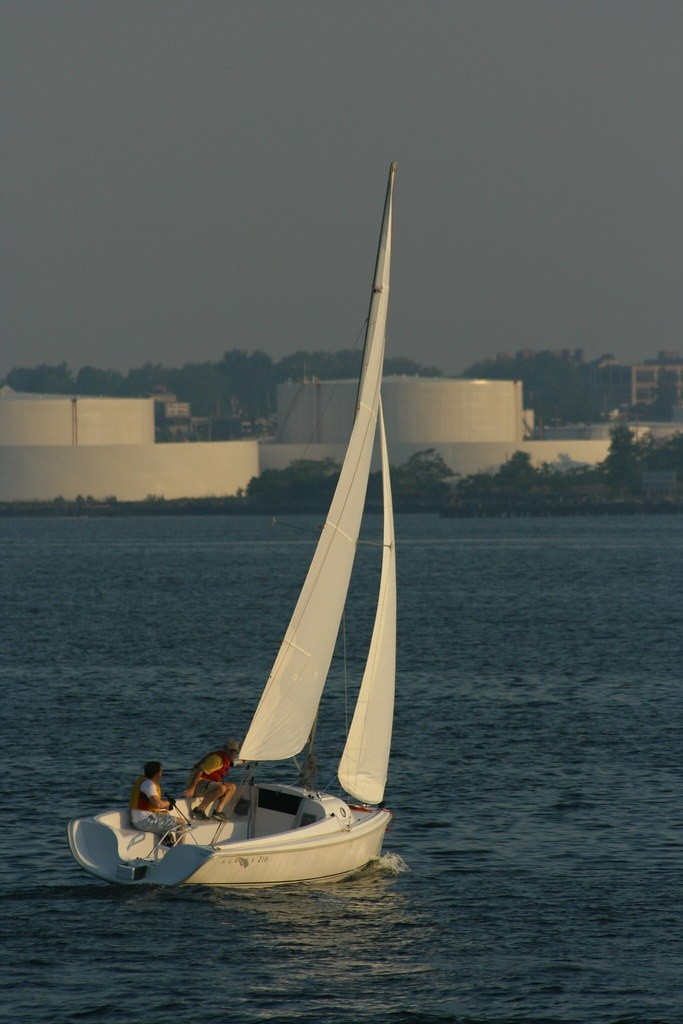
[191,807,211,820]
[212,809,230,821]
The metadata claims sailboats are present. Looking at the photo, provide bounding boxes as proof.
[67,157,403,892]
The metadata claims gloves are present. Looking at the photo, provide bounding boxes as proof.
[167,798,176,806]
[168,803,173,811]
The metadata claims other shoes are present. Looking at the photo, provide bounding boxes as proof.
[160,834,175,847]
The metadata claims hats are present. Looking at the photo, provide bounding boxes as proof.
[224,739,238,751]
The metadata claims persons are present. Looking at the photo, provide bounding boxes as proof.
[129,761,186,847]
[182,739,251,821]
